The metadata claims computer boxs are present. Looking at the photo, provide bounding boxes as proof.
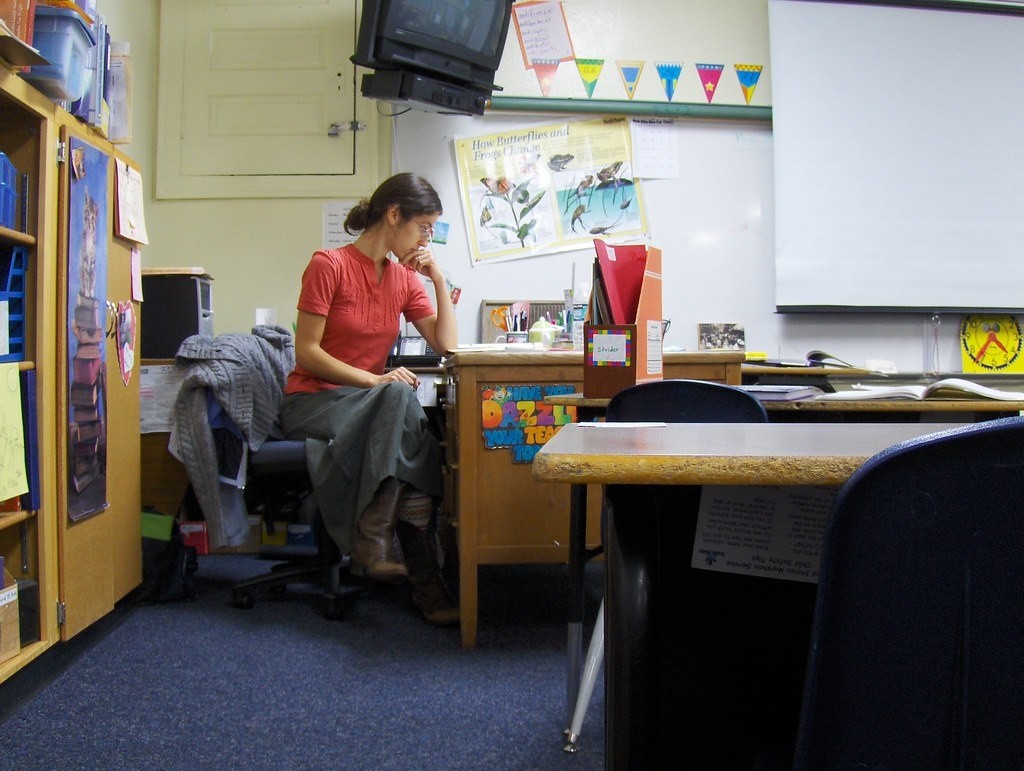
[140,275,213,360]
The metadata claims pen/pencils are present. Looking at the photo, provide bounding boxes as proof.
[511,310,527,332]
[545,309,572,333]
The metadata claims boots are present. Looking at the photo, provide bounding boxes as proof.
[348,476,410,582]
[396,507,461,629]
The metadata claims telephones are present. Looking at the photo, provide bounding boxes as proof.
[387,330,441,366]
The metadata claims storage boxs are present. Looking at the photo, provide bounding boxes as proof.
[0,567,21,664]
[16,5,98,104]
[584,246,664,398]
[0,152,20,230]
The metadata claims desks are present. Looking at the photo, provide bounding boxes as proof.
[549,389,1024,745]
[444,351,747,650]
[743,365,870,393]
[533,422,995,771]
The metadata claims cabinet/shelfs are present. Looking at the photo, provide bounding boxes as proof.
[0,62,145,725]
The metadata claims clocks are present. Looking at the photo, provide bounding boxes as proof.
[960,313,1024,374]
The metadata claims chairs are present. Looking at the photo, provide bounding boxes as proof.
[192,334,357,624]
[790,414,1024,771]
[610,379,769,425]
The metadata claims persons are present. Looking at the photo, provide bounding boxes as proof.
[278,172,463,625]
[700,328,744,349]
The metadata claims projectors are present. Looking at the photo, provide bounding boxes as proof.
[360,72,486,117]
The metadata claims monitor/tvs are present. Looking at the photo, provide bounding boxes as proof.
[373,0,512,84]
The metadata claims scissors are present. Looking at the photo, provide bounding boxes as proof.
[490,306,508,332]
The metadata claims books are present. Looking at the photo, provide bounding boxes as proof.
[764,350,857,368]
[813,378,1023,402]
[724,385,814,400]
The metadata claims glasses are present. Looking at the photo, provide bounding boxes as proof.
[411,218,431,238]
